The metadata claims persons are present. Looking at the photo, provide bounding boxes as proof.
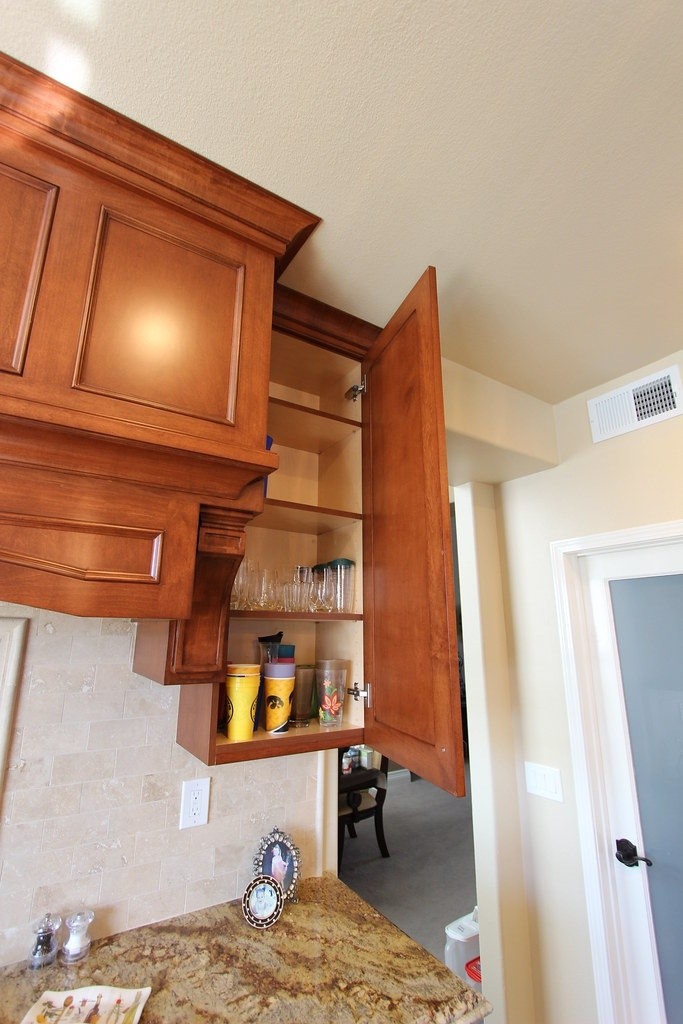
[271,844,290,892]
[251,889,268,916]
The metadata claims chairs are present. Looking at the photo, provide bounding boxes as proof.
[337,750,392,874]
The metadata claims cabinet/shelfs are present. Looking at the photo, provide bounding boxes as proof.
[0,51,469,800]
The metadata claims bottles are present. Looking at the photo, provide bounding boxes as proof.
[61,990,143,1024]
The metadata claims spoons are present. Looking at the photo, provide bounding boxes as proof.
[53,996,74,1023]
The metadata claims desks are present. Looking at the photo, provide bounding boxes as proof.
[1,866,494,1024]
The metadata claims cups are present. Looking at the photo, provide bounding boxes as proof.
[230,555,356,615]
[217,630,349,743]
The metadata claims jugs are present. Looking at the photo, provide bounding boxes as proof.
[444,905,481,995]
